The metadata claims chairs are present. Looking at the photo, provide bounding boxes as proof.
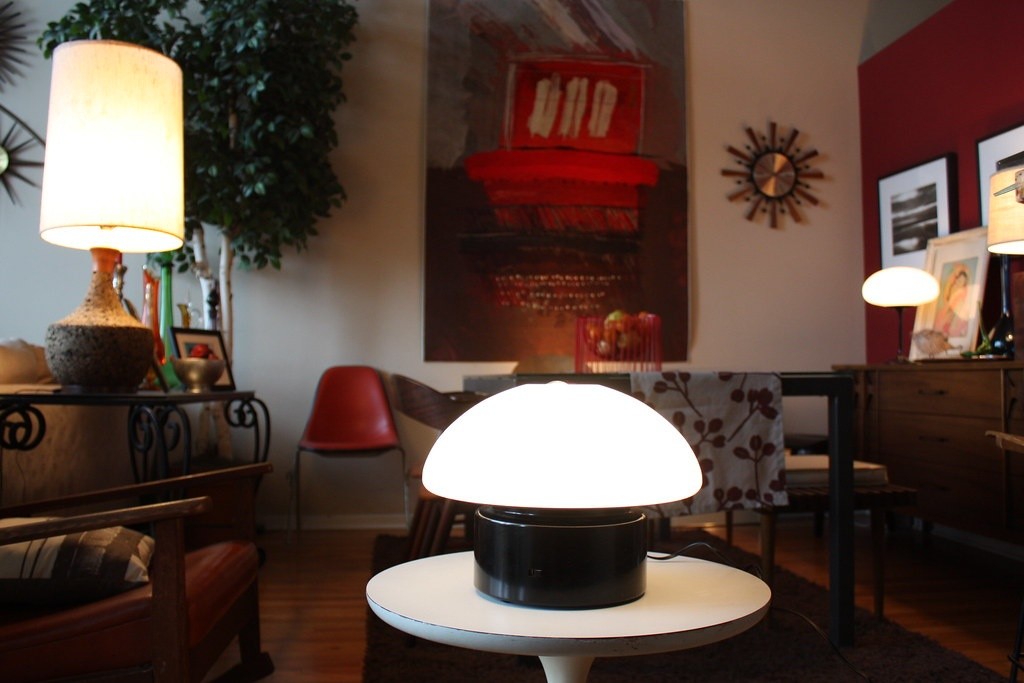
[0,460,275,683]
[284,364,411,546]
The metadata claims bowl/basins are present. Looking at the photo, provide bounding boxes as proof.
[173,360,223,393]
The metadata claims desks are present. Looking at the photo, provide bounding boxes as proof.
[0,388,272,550]
[363,548,771,683]
[373,370,864,652]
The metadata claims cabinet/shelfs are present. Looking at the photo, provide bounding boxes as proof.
[828,355,1024,552]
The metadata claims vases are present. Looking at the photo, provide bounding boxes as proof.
[157,264,185,393]
[142,265,167,393]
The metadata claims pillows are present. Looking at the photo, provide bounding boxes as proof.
[0,515,156,621]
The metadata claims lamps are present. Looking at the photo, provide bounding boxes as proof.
[39,39,187,394]
[987,163,1024,256]
[859,265,939,367]
[421,381,704,610]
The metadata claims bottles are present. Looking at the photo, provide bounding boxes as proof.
[991,254,1015,358]
[142,252,184,392]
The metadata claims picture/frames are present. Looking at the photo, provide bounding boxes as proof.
[901,223,988,361]
[976,121,1024,226]
[875,151,958,274]
[168,326,237,393]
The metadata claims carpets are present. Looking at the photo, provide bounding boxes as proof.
[359,526,1021,683]
